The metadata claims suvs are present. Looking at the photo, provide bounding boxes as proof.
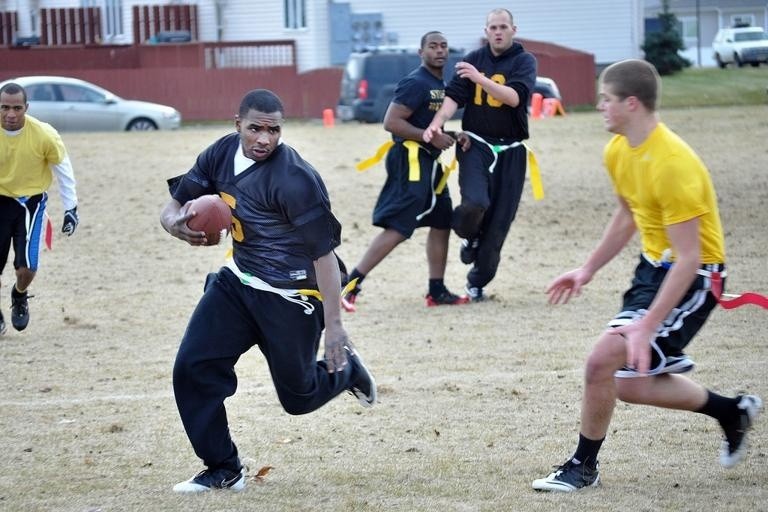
[335,48,561,123]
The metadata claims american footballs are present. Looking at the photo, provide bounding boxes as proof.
[186,195,232,246]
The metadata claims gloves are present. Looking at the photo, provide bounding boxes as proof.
[62,207,80,236]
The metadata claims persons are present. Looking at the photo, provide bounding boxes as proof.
[0,84,79,336]
[530,59,762,491]
[337,32,470,312]
[161,87,377,495]
[423,7,537,299]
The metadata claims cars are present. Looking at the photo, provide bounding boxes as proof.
[712,27,768,69]
[0,74,182,132]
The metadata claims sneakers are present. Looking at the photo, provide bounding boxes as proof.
[10,284,30,332]
[340,275,359,314]
[173,465,249,492]
[719,391,763,467]
[426,290,469,308]
[532,456,601,492]
[341,339,376,409]
[464,275,485,302]
[461,237,478,263]
